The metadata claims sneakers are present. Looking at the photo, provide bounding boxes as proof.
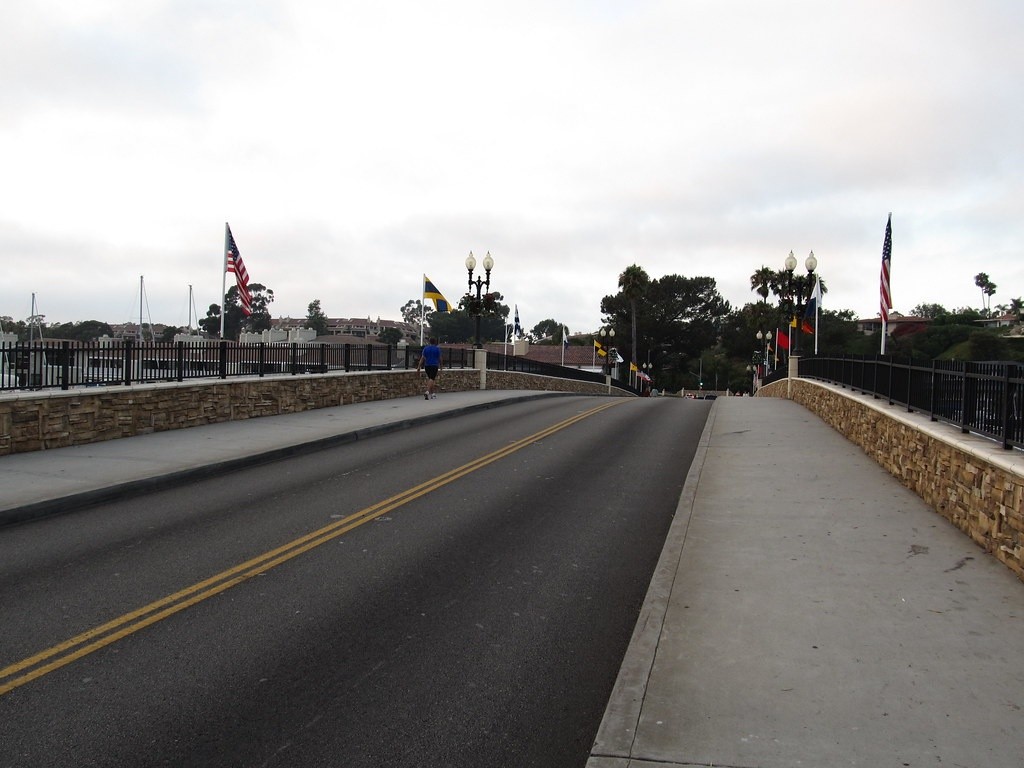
[431,393,437,399]
[424,391,430,400]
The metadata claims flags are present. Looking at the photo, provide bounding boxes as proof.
[227,229,253,316]
[777,330,793,354]
[515,309,521,336]
[616,352,624,363]
[759,366,763,373]
[802,279,821,334]
[879,217,893,324]
[637,369,643,377]
[564,331,569,348]
[631,363,638,371]
[769,343,780,362]
[424,276,452,312]
[595,342,607,359]
[788,300,797,327]
[643,373,651,380]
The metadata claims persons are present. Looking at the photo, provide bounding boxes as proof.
[418,337,444,399]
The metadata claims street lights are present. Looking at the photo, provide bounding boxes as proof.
[464,248,496,389]
[747,365,757,394]
[756,329,773,378]
[643,362,653,397]
[599,327,616,395]
[783,248,818,358]
